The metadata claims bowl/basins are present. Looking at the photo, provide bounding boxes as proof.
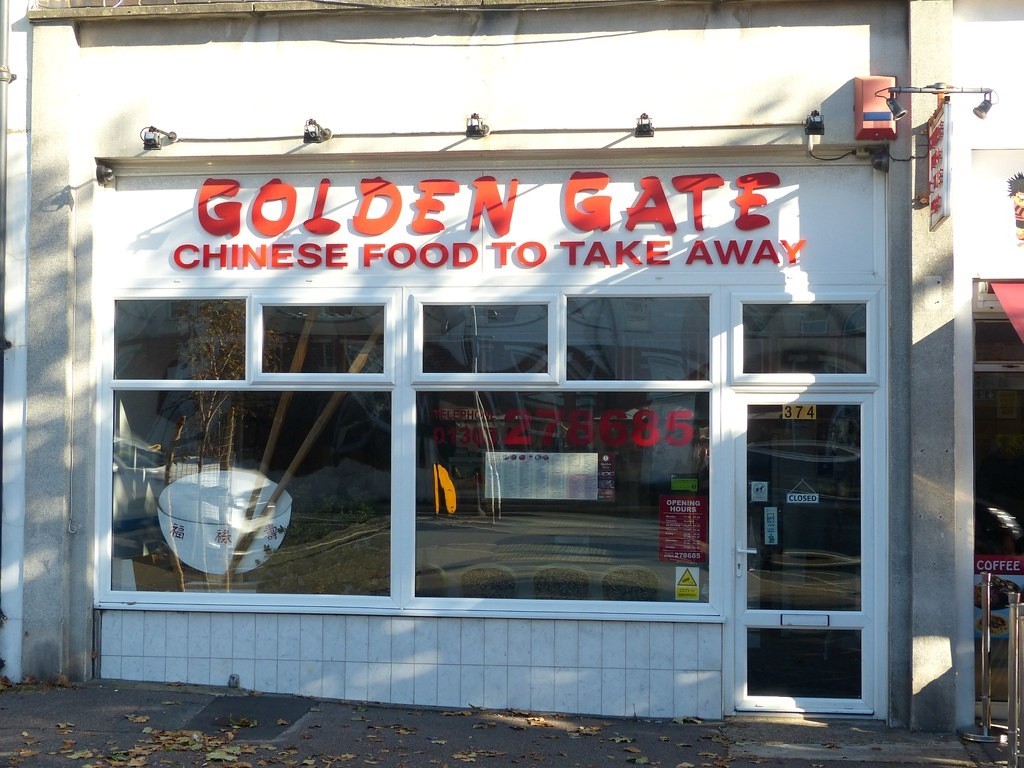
[158,471,292,575]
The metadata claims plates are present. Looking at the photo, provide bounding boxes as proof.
[975,613,1009,636]
[974,582,1022,614]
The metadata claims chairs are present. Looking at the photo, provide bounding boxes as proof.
[416,561,663,600]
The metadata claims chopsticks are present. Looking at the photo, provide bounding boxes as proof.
[222,307,384,583]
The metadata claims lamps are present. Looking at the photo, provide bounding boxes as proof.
[466,112,491,137]
[302,117,332,143]
[634,112,655,137]
[804,109,824,135]
[874,84,994,119]
[140,125,177,150]
